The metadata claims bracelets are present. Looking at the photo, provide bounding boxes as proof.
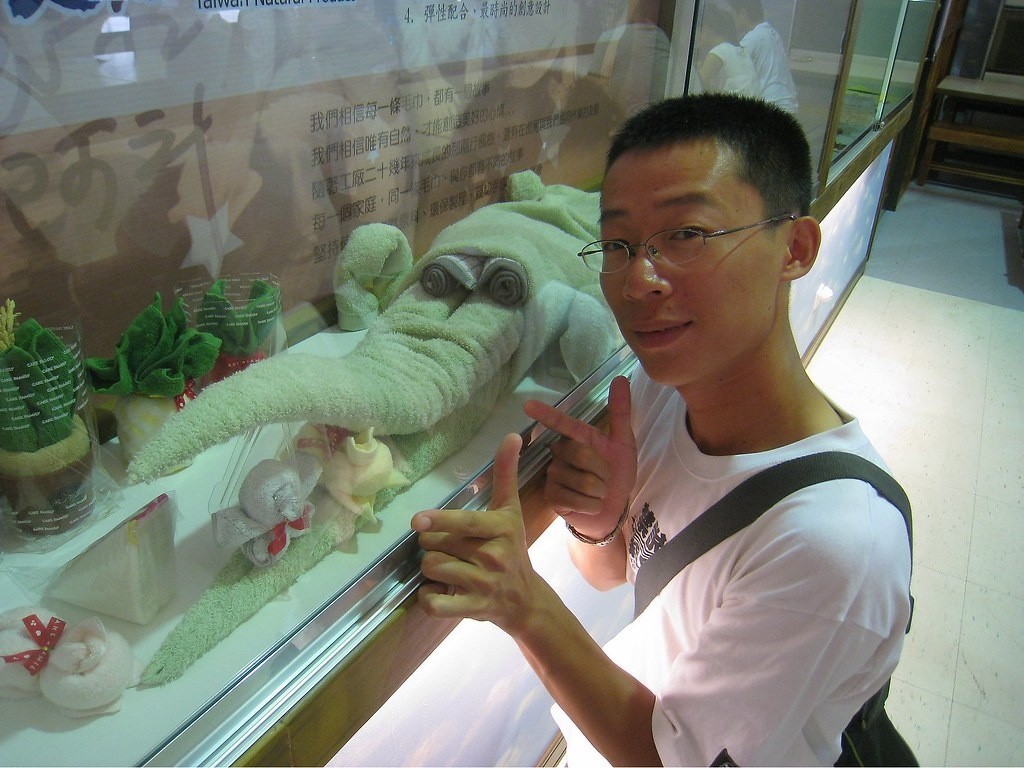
[565,498,632,548]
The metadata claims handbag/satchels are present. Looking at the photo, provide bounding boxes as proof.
[637,452,921,768]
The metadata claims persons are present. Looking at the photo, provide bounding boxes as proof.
[729,0,799,117]
[696,6,760,97]
[408,91,919,768]
[586,0,685,108]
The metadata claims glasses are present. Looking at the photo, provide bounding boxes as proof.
[578,213,798,274]
